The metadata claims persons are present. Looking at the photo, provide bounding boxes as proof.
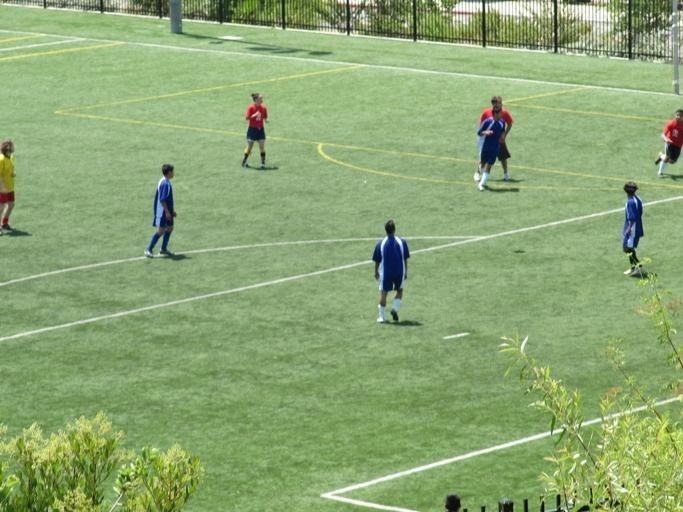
[622,181,645,277]
[480,95,513,181]
[241,92,269,168]
[1,137,18,232]
[145,163,177,258]
[372,218,410,323]
[473,104,506,191]
[654,109,683,178]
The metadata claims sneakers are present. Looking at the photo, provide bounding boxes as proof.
[241,161,268,173]
[622,266,649,277]
[653,153,665,180]
[0,223,16,236]
[145,248,173,260]
[503,174,512,182]
[473,171,490,192]
[376,310,401,325]
